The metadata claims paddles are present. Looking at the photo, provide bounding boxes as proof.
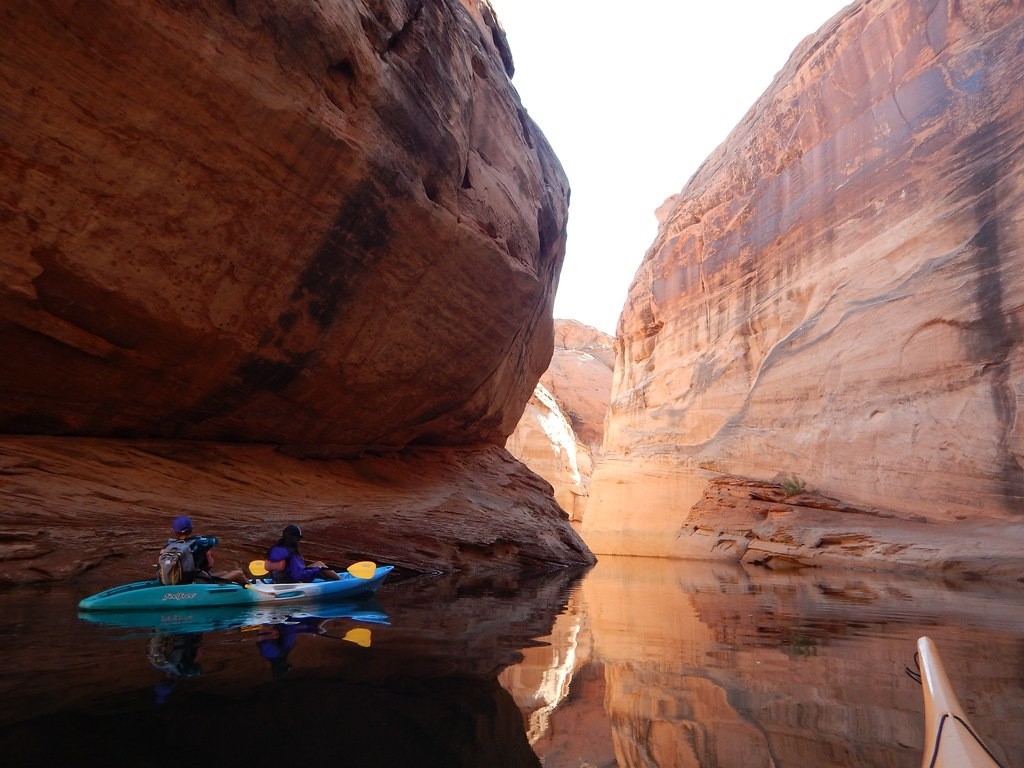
[248,560,376,580]
[152,563,279,597]
[242,625,372,650]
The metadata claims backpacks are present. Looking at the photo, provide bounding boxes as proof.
[158,539,197,585]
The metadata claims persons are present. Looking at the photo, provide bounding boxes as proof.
[265,525,344,584]
[166,517,255,589]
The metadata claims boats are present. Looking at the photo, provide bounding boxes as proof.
[78,598,391,632]
[78,565,394,610]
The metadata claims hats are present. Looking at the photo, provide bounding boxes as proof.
[172,516,192,533]
[285,525,304,539]
